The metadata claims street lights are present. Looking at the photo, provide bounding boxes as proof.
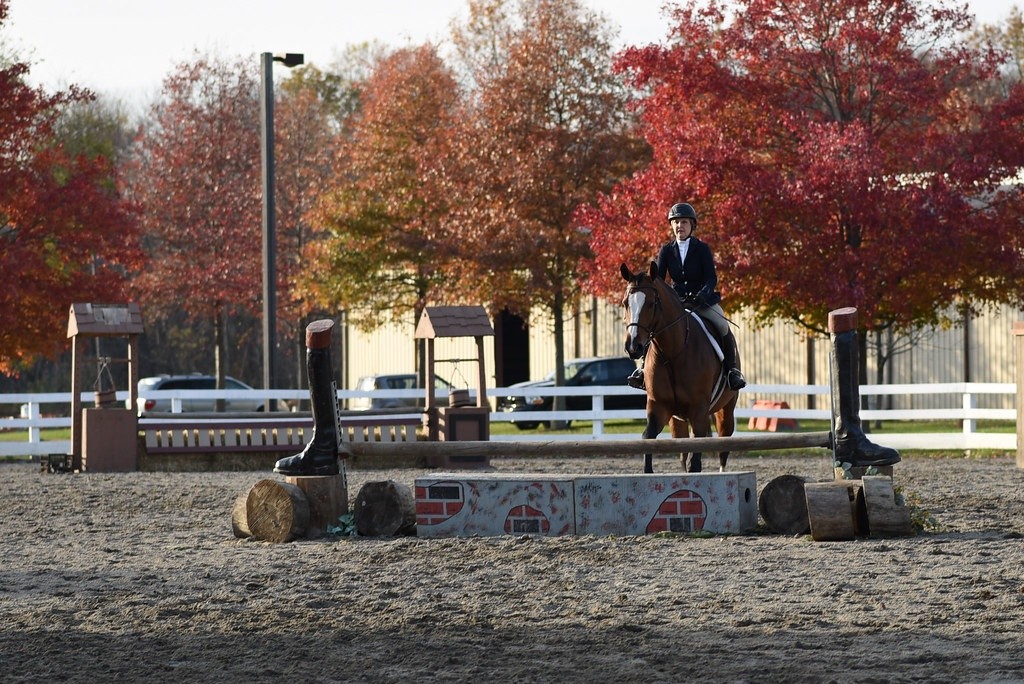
[260,52,304,413]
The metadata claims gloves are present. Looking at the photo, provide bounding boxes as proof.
[693,294,704,303]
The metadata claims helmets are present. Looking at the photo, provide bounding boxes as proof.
[669,203,697,224]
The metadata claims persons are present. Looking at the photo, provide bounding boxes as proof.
[621,202,747,391]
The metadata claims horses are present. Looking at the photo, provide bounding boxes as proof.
[621,260,742,475]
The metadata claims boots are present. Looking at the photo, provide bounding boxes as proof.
[627,358,645,389]
[829,331,901,464]
[273,346,340,474]
[719,331,746,389]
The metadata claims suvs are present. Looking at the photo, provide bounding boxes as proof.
[125,372,289,412]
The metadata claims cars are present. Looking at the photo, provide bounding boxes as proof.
[350,373,492,412]
[499,357,647,430]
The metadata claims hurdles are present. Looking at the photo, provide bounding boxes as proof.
[273,304,904,543]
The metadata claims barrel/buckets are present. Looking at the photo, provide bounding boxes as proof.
[448,382,470,407]
[92,381,117,408]
